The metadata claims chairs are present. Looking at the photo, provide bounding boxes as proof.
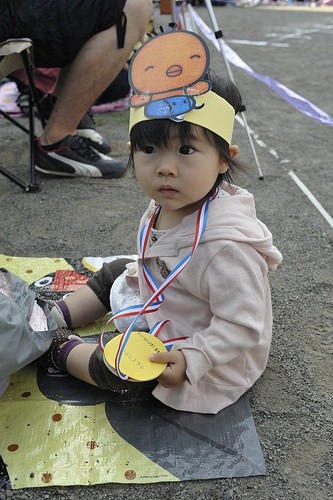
[0,38,47,193]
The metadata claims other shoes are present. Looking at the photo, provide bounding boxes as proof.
[34,298,64,329]
[43,329,74,368]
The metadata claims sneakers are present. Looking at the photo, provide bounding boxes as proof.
[33,134,124,178]
[33,93,111,154]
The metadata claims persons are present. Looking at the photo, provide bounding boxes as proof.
[34,22,286,415]
[0,0,154,179]
